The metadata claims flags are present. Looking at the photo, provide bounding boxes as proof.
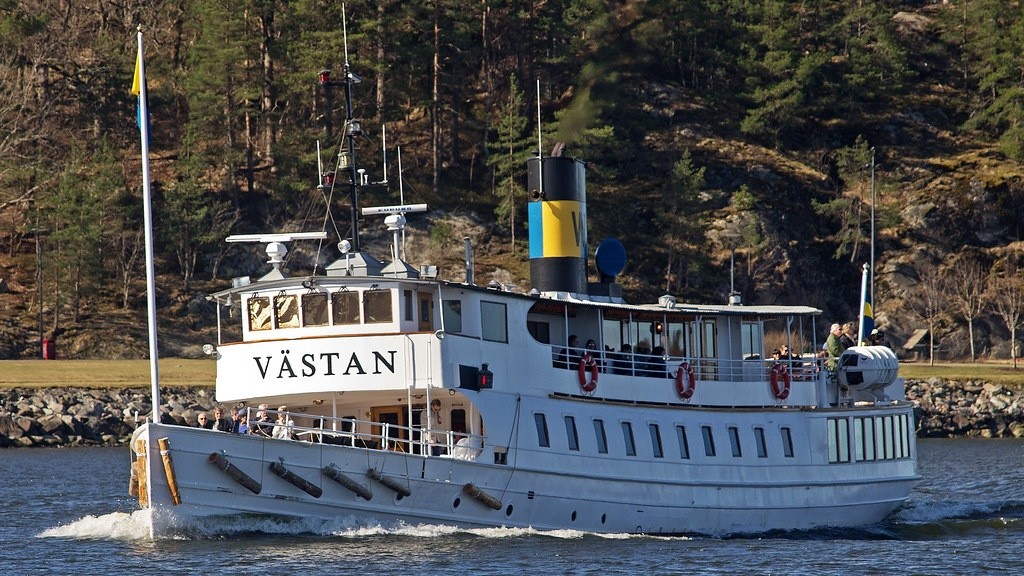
[131,53,152,146]
[861,274,874,342]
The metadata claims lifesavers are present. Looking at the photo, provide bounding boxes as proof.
[579,355,599,391]
[677,362,695,398]
[770,364,790,399]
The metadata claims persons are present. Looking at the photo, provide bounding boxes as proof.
[773,345,802,372]
[817,322,856,373]
[635,346,673,378]
[252,404,293,438]
[421,399,464,456]
[559,335,607,372]
[614,344,631,375]
[195,407,247,434]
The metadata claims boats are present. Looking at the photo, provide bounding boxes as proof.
[129,2,925,544]
